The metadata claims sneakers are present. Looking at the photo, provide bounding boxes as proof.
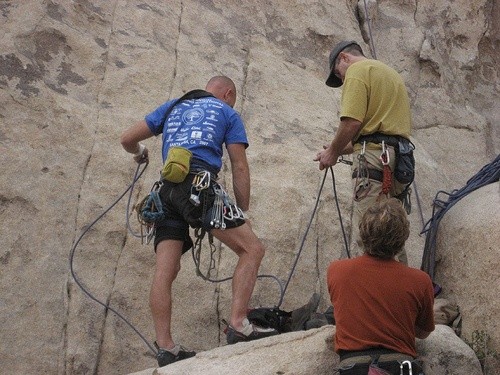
[153,341,196,367]
[220,317,279,344]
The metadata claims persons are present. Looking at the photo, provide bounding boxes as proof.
[327,198,435,375]
[312,41,415,326]
[120,76,278,367]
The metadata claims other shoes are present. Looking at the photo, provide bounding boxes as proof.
[432,280,442,298]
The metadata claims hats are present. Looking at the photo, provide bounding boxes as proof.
[326,40,363,88]
